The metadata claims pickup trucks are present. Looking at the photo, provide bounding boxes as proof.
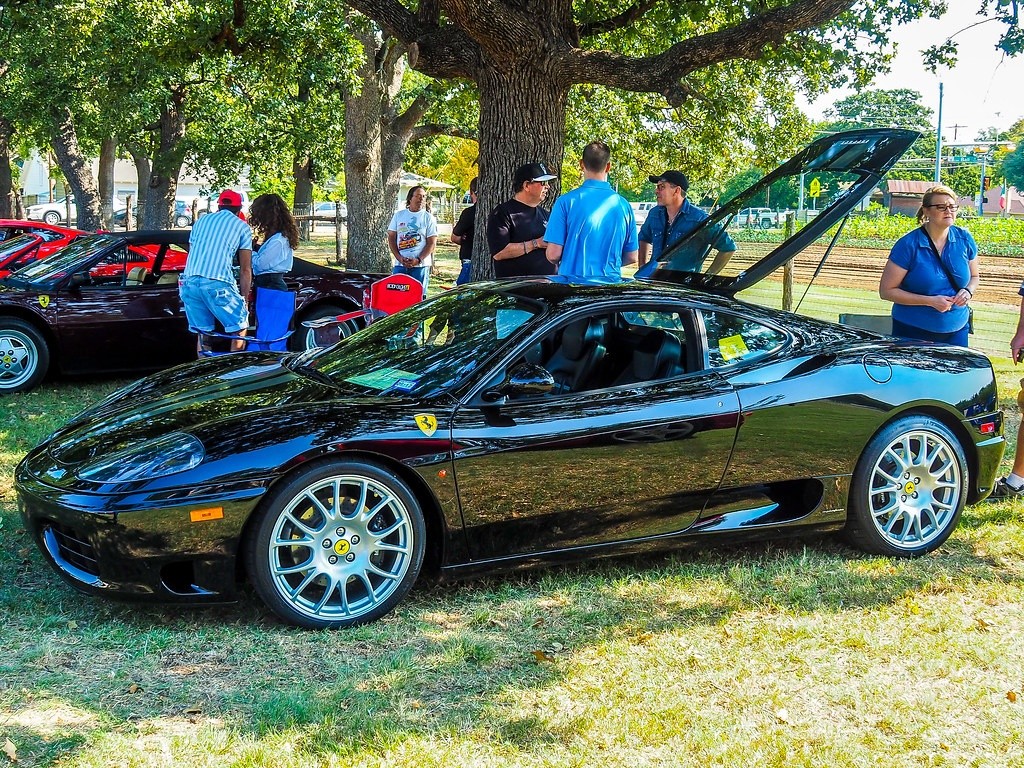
[631,200,658,224]
[194,191,260,222]
[736,207,787,228]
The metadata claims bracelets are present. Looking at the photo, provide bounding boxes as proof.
[532,239,538,250]
[964,288,972,299]
[523,241,528,254]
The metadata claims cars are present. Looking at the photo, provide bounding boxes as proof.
[0,218,195,284]
[310,201,349,220]
[13,127,1005,629]
[0,229,395,394]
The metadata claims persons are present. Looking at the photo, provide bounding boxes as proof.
[987,281,1024,499]
[542,143,639,277]
[249,192,300,352]
[178,188,249,359]
[879,184,979,349]
[386,185,438,300]
[486,163,559,277]
[451,177,479,287]
[637,170,737,275]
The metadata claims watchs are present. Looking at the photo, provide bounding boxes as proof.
[417,256,423,264]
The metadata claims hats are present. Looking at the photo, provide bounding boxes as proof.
[218,189,242,206]
[515,162,558,182]
[650,170,691,192]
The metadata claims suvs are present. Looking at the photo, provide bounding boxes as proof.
[24,190,127,223]
[114,200,192,227]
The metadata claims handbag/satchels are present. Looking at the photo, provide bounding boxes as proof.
[966,307,974,334]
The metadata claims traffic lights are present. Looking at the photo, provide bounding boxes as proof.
[983,177,990,192]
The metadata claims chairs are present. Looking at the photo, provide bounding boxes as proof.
[157,272,179,284]
[575,325,682,391]
[121,267,147,286]
[537,319,604,398]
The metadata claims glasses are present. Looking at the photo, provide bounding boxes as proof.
[528,180,549,187]
[925,204,960,213]
[655,184,678,191]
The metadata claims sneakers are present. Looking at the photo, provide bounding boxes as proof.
[986,476,1024,503]
